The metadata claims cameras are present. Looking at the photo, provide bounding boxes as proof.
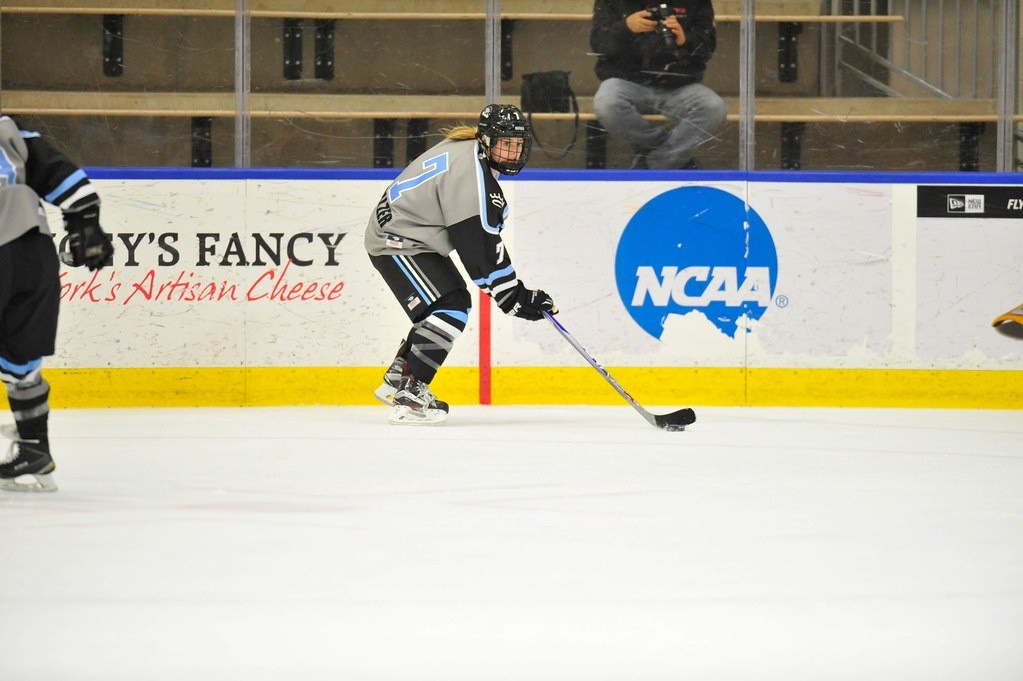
[643,3,676,48]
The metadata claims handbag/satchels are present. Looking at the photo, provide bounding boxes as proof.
[521,71,580,159]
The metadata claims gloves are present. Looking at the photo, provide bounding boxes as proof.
[62,206,115,272]
[500,279,560,321]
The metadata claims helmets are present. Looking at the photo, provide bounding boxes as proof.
[475,104,532,177]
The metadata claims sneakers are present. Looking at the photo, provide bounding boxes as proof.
[388,363,448,423]
[375,339,409,406]
[0,439,58,491]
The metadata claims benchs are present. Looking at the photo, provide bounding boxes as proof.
[0,0,903,86]
[0,90,1023,170]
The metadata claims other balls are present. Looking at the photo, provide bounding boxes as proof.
[667,423,686,432]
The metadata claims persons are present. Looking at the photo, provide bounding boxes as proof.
[0,114,114,494]
[588,0,727,169]
[365,103,559,427]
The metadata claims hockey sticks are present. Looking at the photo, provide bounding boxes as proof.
[540,309,697,429]
